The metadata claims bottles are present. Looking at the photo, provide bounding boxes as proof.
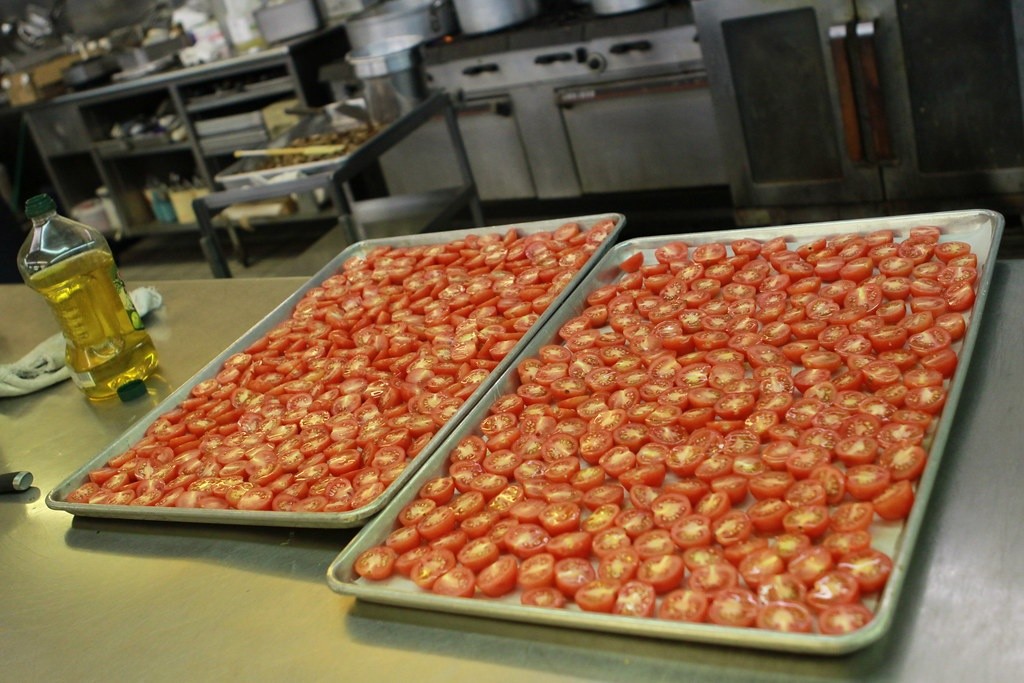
[98,187,122,230]
[18,194,160,401]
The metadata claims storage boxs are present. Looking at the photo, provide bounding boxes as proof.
[166,188,210,224]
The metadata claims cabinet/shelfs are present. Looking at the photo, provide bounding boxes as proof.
[15,16,351,267]
[190,91,482,279]
[693,0,1023,231]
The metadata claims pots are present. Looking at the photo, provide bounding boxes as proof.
[593,0,653,15]
[454,0,538,34]
[346,0,445,44]
[63,54,122,92]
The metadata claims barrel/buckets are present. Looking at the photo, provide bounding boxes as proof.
[73,199,111,233]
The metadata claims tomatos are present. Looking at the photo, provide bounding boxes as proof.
[354,226,978,634]
[65,220,615,513]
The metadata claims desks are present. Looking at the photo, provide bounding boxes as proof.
[0,260,1024,682]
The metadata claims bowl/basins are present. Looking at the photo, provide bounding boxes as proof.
[346,35,424,78]
